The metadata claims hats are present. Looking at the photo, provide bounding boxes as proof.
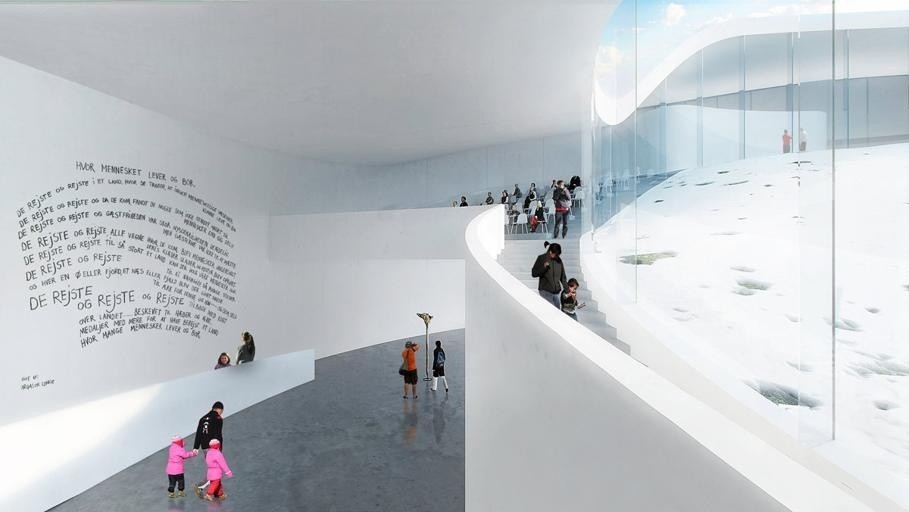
[172,433,183,448]
[406,341,412,346]
[209,438,221,448]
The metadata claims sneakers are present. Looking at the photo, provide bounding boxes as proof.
[193,484,226,502]
[168,491,185,498]
[403,396,417,400]
[431,388,448,392]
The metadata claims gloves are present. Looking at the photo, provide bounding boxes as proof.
[226,471,232,477]
[192,449,198,455]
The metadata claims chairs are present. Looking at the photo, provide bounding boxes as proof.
[506,166,640,235]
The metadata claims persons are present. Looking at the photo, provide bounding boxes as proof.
[563,277,582,321]
[401,342,421,400]
[450,175,585,238]
[533,241,567,312]
[166,436,199,499]
[783,130,792,153]
[432,341,450,394]
[237,332,258,363]
[201,439,234,501]
[193,402,226,497]
[215,352,233,370]
[800,127,808,153]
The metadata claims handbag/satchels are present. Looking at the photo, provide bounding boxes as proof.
[399,360,408,375]
